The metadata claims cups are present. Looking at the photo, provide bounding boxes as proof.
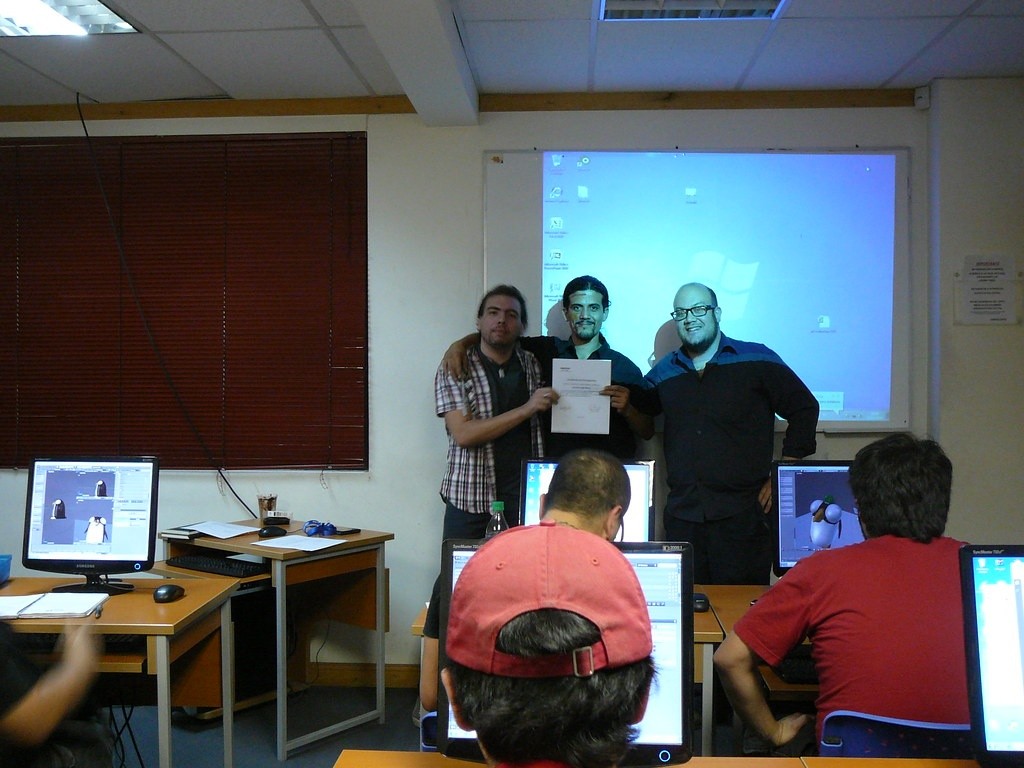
[258,498,277,523]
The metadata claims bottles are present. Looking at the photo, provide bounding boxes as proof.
[485,502,509,542]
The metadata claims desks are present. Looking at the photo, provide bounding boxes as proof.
[332,749,983,768]
[146,517,394,761]
[410,584,822,757]
[0,577,241,768]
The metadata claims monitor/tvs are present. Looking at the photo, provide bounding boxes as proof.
[771,457,866,578]
[440,455,693,768]
[21,453,159,595]
[959,542,1024,768]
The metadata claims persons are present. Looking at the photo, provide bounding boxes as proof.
[643,282,819,754]
[0,612,116,768]
[442,256,655,464]
[437,515,658,768]
[536,450,630,551]
[411,286,560,750]
[711,432,977,753]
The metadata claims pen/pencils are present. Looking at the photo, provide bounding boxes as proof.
[95,604,102,618]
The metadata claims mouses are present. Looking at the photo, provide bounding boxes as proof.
[259,526,289,536]
[693,593,709,611]
[153,584,185,603]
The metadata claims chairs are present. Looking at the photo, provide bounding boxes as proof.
[821,710,972,760]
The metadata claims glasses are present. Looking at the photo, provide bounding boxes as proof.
[671,304,716,322]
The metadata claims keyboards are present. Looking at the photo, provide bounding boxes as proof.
[166,554,272,578]
[15,630,147,654]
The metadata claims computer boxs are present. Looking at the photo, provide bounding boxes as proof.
[194,587,279,712]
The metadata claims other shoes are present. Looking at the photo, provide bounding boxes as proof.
[412,698,419,728]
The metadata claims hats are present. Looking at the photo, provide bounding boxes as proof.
[443,520,651,679]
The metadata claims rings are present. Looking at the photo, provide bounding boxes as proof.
[544,394,548,398]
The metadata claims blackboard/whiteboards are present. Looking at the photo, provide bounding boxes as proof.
[483,147,915,433]
